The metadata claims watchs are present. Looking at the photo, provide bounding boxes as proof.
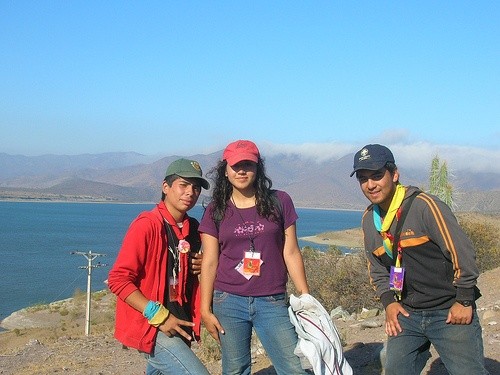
[456,300,472,307]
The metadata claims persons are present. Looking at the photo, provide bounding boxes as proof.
[350,144,491,375]
[197,140,314,375]
[107,158,212,375]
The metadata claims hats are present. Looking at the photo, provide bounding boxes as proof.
[166,159,210,190]
[223,140,259,166]
[349,144,395,177]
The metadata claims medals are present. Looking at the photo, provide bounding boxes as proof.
[178,240,190,254]
